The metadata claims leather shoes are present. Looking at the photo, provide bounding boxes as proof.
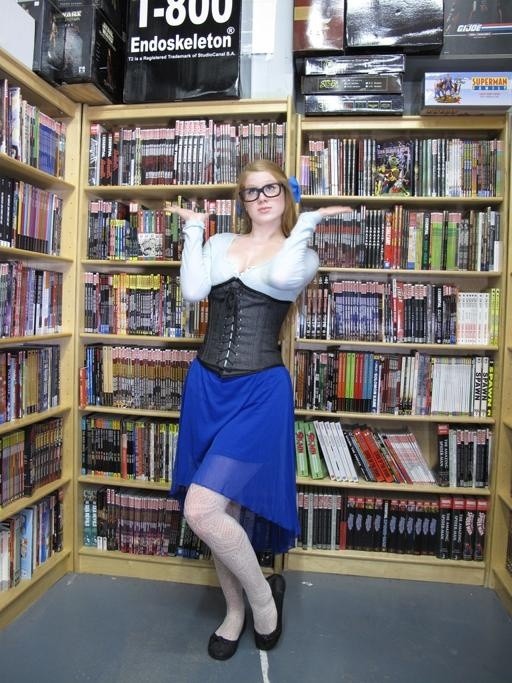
[253,573,286,651]
[207,611,247,662]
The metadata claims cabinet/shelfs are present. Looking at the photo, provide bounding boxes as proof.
[1,46,80,630]
[282,112,511,591]
[75,97,293,589]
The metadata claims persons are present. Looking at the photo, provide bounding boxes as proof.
[168,157,359,662]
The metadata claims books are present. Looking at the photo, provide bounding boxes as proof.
[81,413,178,483]
[1,417,64,509]
[82,485,212,560]
[300,205,502,271]
[87,120,287,186]
[0,179,62,256]
[82,273,210,339]
[1,261,63,339]
[296,137,502,198]
[296,486,490,561]
[293,345,495,417]
[292,419,493,489]
[86,195,251,261]
[1,80,66,180]
[1,489,64,593]
[79,342,201,411]
[1,344,61,424]
[299,273,500,346]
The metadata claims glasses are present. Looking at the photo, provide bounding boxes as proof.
[238,182,284,203]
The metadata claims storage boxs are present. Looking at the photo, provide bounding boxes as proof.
[34,1,125,103]
[413,70,512,112]
[302,53,407,76]
[300,72,403,94]
[304,94,404,115]
[344,1,445,53]
